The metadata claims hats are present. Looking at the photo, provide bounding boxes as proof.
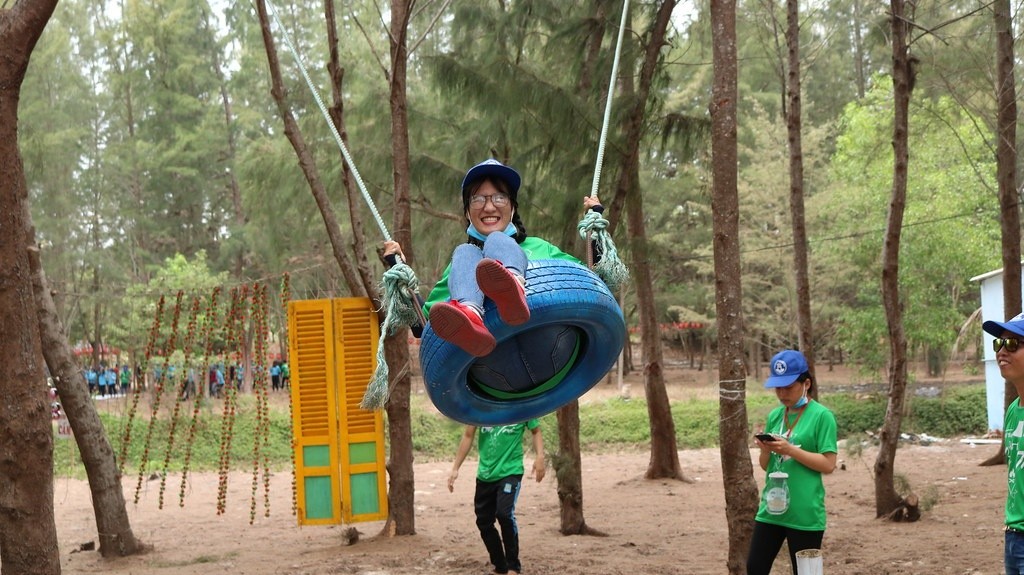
[110,368,113,371]
[461,158,521,193]
[764,350,808,387]
[982,312,1024,339]
[122,365,128,367]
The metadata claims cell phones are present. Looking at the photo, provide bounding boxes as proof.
[755,434,776,443]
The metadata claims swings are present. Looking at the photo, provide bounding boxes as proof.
[263,0,632,429]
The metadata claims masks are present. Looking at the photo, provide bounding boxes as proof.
[792,383,808,408]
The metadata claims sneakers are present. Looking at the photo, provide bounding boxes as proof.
[476,257,530,326]
[429,299,497,357]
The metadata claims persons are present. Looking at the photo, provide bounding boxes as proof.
[85,360,290,399]
[374,154,606,399]
[744,345,841,575]
[979,310,1024,575]
[446,417,547,574]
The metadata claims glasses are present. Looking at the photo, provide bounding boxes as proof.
[468,192,511,210]
[993,337,1024,352]
